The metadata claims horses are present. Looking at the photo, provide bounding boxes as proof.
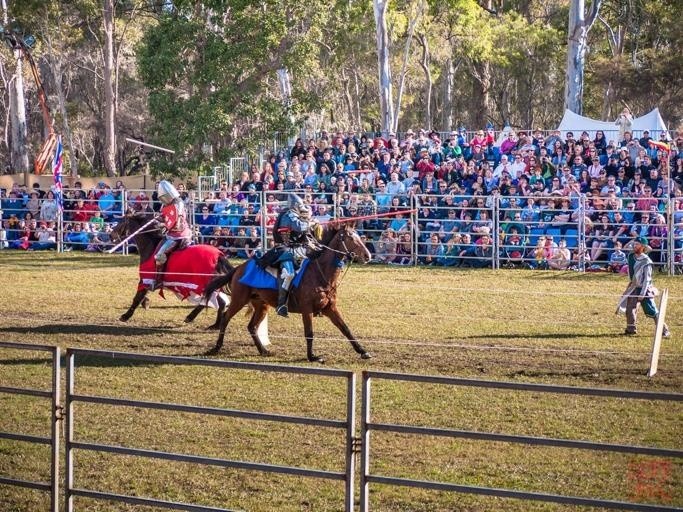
[196,219,375,363]
[107,208,235,332]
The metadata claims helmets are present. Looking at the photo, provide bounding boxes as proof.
[287,192,304,208]
[157,180,180,205]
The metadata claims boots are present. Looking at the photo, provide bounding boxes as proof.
[276,274,292,317]
[149,261,166,291]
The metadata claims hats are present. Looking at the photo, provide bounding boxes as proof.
[634,236,648,245]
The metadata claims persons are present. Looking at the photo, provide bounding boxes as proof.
[144,179,194,291]
[616,237,670,338]
[272,192,321,316]
[176,130,682,271]
[0,181,161,254]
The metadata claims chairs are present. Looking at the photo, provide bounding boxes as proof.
[523,228,577,255]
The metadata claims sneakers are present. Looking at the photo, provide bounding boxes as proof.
[625,328,637,334]
[662,328,670,337]
